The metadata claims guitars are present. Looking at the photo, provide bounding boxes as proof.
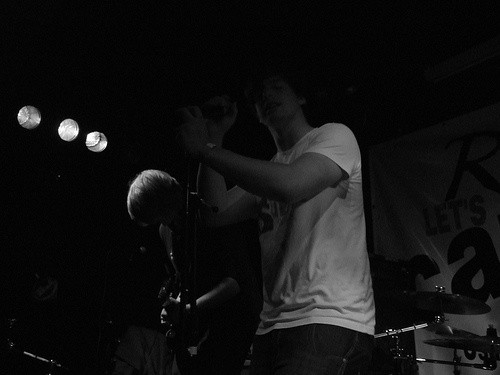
[157,285,181,338]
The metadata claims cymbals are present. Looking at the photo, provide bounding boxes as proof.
[372,254,440,281]
[391,290,493,316]
[423,335,500,353]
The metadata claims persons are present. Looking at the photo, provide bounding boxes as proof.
[172,65,377,375]
[111,222,179,375]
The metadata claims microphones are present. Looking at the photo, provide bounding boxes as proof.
[199,106,228,120]
